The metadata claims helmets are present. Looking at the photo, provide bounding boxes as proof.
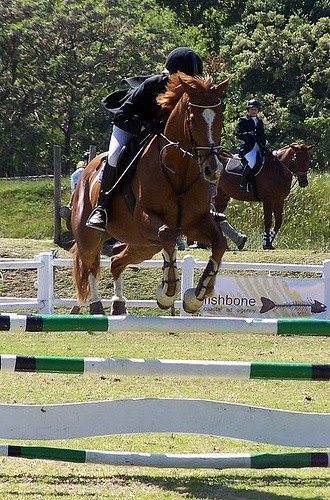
[164,47,203,76]
[245,99,262,113]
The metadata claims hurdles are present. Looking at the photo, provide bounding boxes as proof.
[0,252,330,316]
[1,313,329,470]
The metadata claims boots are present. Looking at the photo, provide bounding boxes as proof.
[85,164,118,234]
[239,164,253,188]
[210,209,227,222]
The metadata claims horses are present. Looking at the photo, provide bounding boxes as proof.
[71,70,230,315]
[212,140,316,249]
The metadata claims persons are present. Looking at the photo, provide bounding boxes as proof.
[71,47,247,250]
[234,99,269,193]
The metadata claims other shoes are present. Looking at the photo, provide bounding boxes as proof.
[236,235,248,249]
